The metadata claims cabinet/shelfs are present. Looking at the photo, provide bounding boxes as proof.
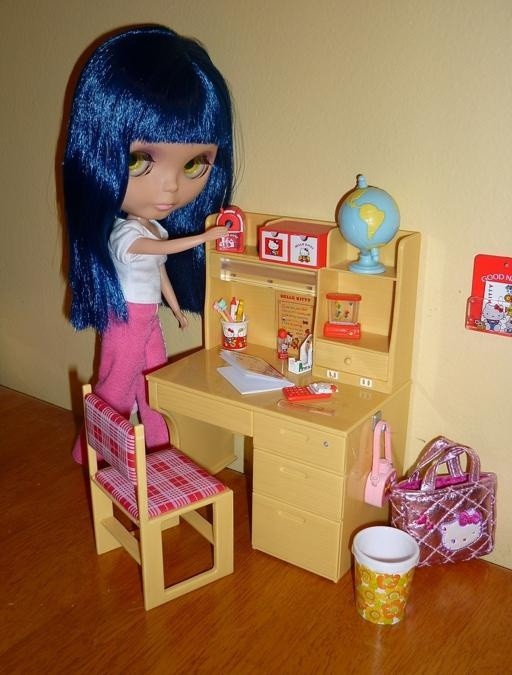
[145,210,423,582]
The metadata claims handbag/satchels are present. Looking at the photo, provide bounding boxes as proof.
[363,419,398,508]
[388,436,498,568]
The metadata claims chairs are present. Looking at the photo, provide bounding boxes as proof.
[79,381,233,611]
